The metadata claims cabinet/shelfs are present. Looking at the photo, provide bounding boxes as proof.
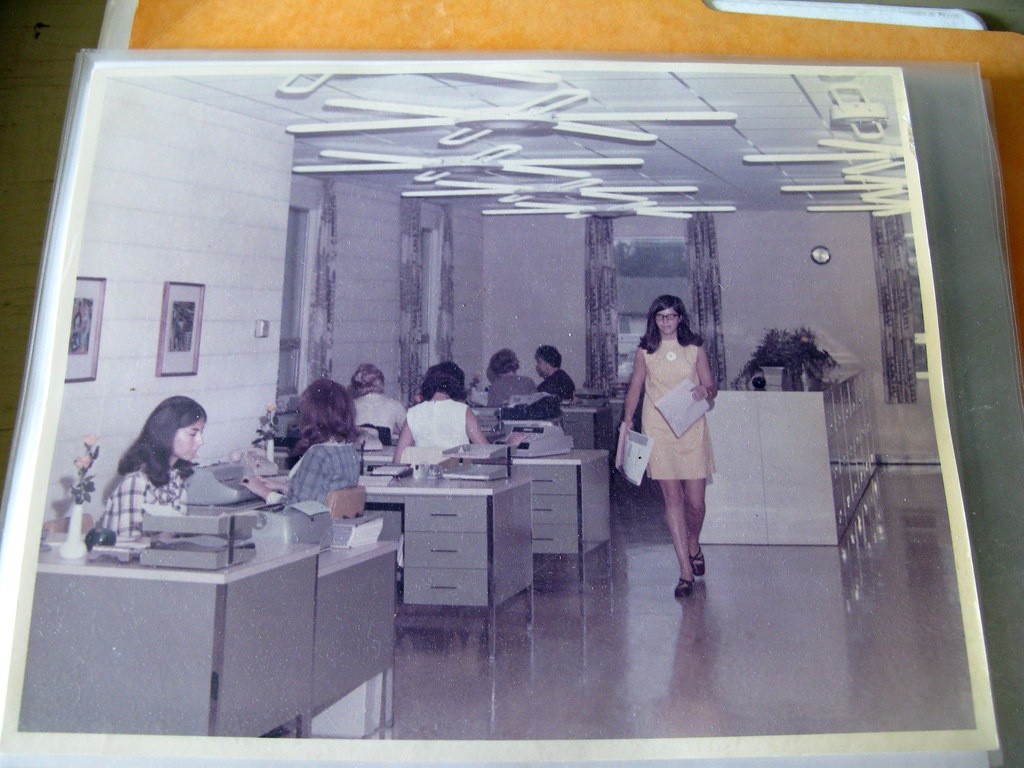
[701,365,879,546]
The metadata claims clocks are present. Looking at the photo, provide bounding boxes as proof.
[810,245,831,264]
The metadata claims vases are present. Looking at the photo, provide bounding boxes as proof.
[59,504,87,559]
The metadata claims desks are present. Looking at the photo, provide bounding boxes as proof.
[17,398,624,739]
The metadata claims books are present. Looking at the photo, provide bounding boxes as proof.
[616,423,654,486]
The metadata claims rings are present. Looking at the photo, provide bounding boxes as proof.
[243,479,248,483]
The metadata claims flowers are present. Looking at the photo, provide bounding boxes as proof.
[70,433,101,506]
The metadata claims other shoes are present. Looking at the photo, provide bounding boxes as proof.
[674,577,694,597]
[689,547,705,576]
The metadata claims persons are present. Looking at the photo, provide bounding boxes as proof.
[623,295,717,597]
[487,349,534,408]
[103,396,206,540]
[352,363,407,434]
[534,346,574,396]
[72,302,91,354]
[392,362,488,465]
[239,378,361,506]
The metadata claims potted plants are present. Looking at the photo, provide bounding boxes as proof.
[731,325,841,388]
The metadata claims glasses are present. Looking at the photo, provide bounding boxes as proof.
[654,312,679,320]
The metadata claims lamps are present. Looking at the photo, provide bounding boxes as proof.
[280,71,910,218]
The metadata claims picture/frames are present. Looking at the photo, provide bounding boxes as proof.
[154,281,205,377]
[64,275,110,387]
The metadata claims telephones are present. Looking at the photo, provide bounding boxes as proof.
[227,445,281,477]
